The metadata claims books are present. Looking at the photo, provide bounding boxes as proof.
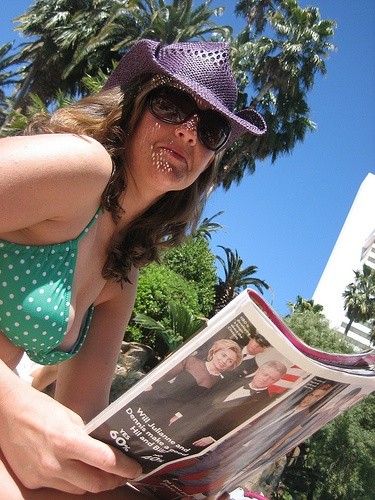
[80,287,375,500]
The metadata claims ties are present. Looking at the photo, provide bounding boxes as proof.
[239,351,247,364]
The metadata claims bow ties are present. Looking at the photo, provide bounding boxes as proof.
[244,383,262,397]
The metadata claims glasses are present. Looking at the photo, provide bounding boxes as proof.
[141,84,233,156]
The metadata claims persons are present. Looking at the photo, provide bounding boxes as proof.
[0,35,270,499]
[160,358,286,456]
[158,380,331,489]
[210,334,272,394]
[131,337,244,442]
[221,386,362,491]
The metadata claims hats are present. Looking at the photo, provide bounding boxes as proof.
[253,332,271,349]
[91,32,268,148]
